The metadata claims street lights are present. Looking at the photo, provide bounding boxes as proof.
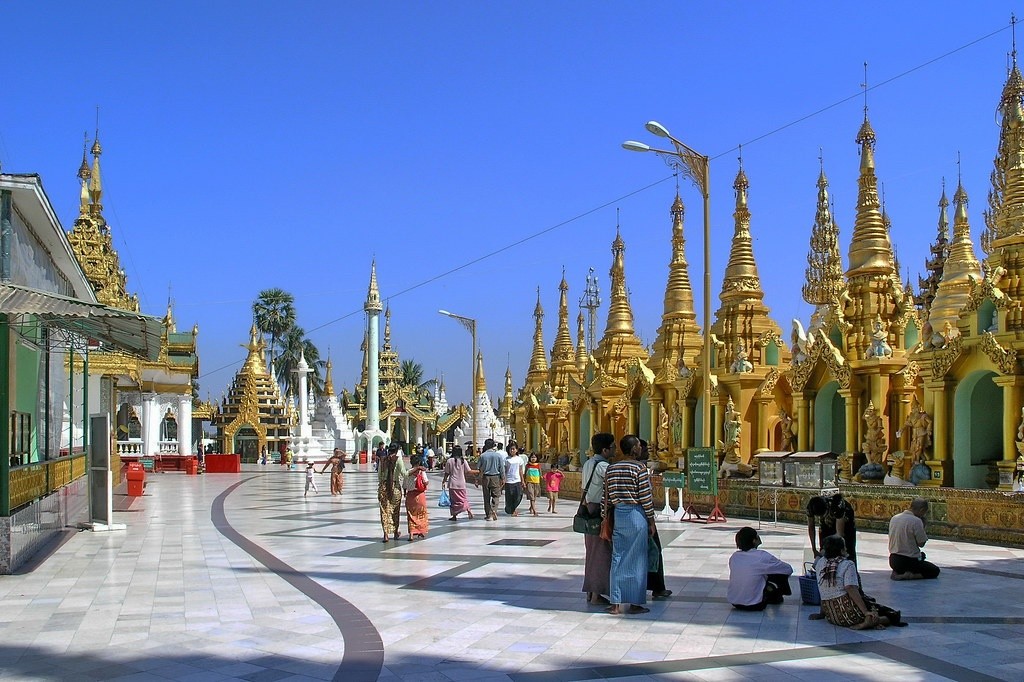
[439,310,476,456]
[622,121,711,447]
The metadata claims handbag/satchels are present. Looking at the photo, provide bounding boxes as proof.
[573,505,608,535]
[416,470,427,492]
[438,484,452,507]
[340,456,345,469]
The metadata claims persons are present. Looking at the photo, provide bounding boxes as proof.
[504,443,526,516]
[403,455,429,540]
[304,460,322,495]
[600,435,656,614]
[543,463,565,514]
[582,433,618,604]
[728,527,793,611]
[889,499,941,580]
[806,494,858,570]
[442,445,480,521]
[815,535,901,630]
[198,444,213,473]
[261,444,266,465]
[637,437,672,596]
[475,439,506,521]
[322,448,346,496]
[378,443,426,541]
[375,440,516,471]
[524,452,543,516]
[284,447,294,469]
